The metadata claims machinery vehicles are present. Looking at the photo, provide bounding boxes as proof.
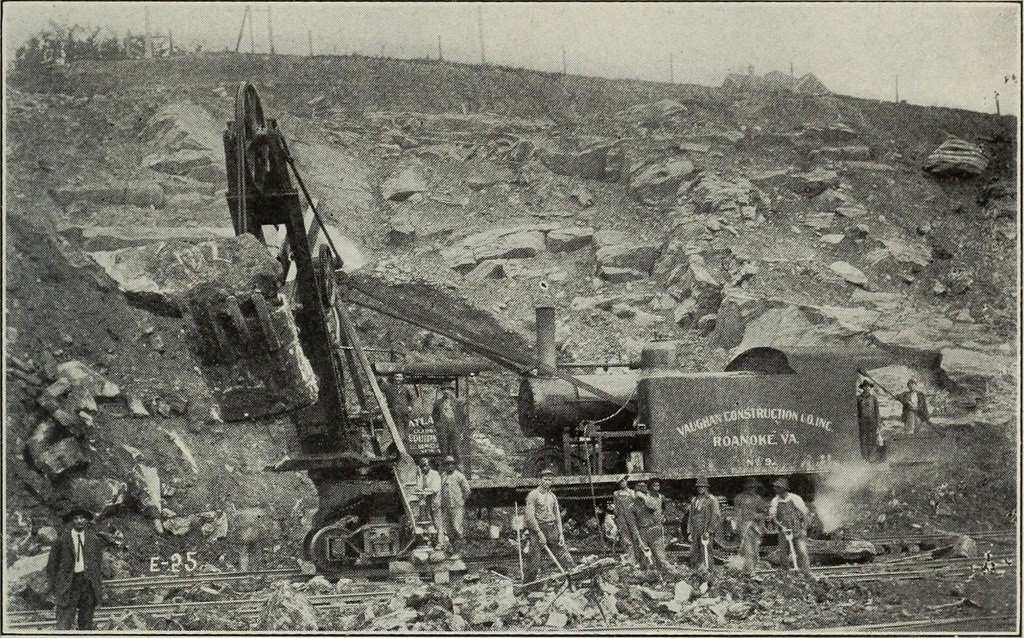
[169,75,978,579]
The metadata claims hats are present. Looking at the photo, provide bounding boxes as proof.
[694,475,711,488]
[614,474,629,484]
[858,380,875,388]
[772,478,791,491]
[443,456,457,464]
[62,504,95,523]
[634,483,648,494]
[907,378,917,387]
[440,381,455,390]
[744,476,762,487]
[539,470,555,479]
[647,476,663,490]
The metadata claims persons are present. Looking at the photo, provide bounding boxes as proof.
[769,478,812,578]
[645,476,669,556]
[45,504,104,631]
[733,475,768,581]
[612,476,643,563]
[893,377,931,437]
[413,457,444,552]
[684,471,720,574]
[437,455,471,554]
[521,469,579,591]
[631,480,672,570]
[856,379,881,465]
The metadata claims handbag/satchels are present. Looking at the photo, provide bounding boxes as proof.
[876,428,884,446]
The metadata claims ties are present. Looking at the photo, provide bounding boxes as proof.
[77,533,84,562]
